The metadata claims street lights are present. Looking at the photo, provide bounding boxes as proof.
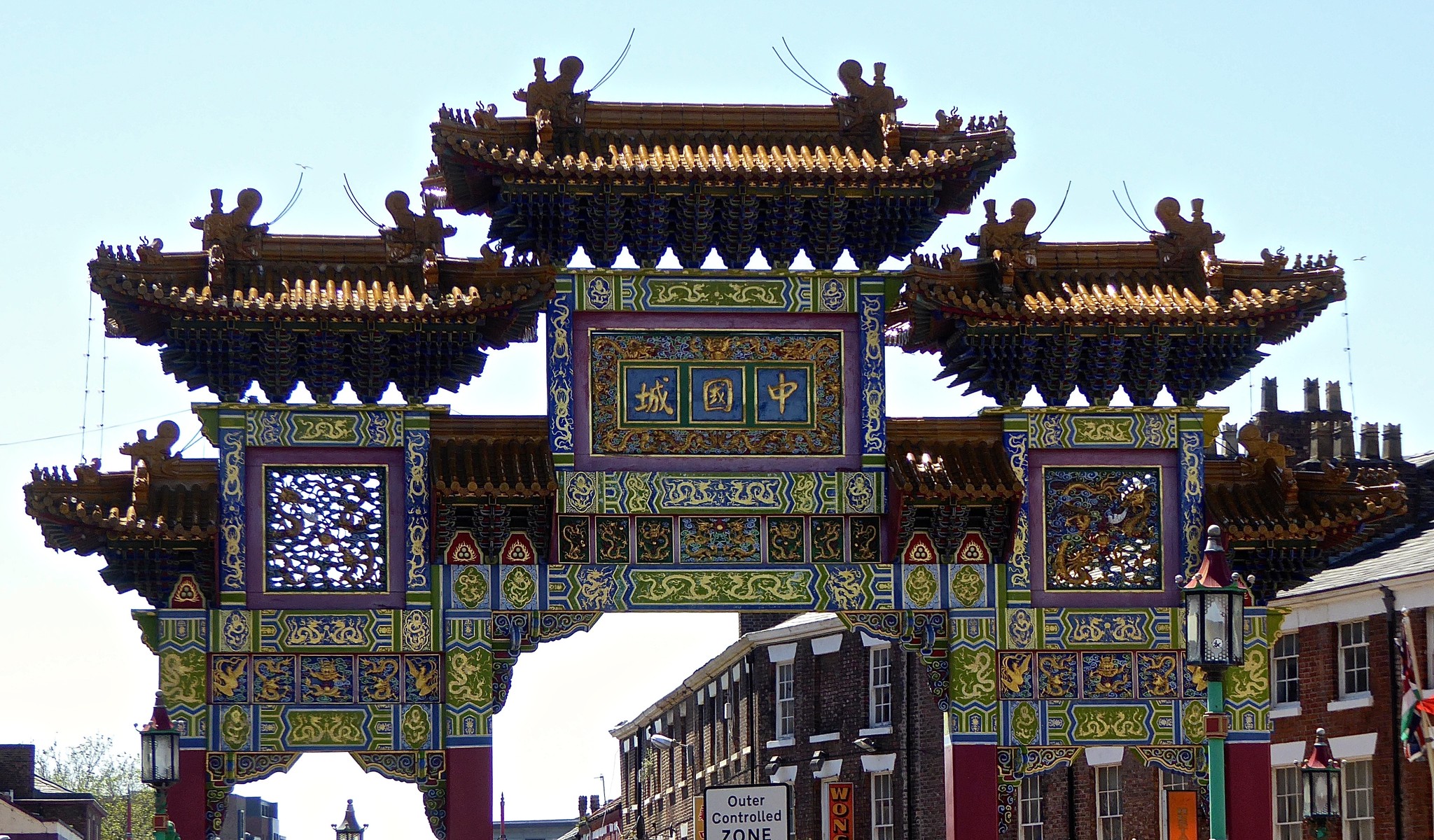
[650,733,697,840]
[1174,526,1257,840]
[1294,728,1348,840]
[133,689,180,840]
